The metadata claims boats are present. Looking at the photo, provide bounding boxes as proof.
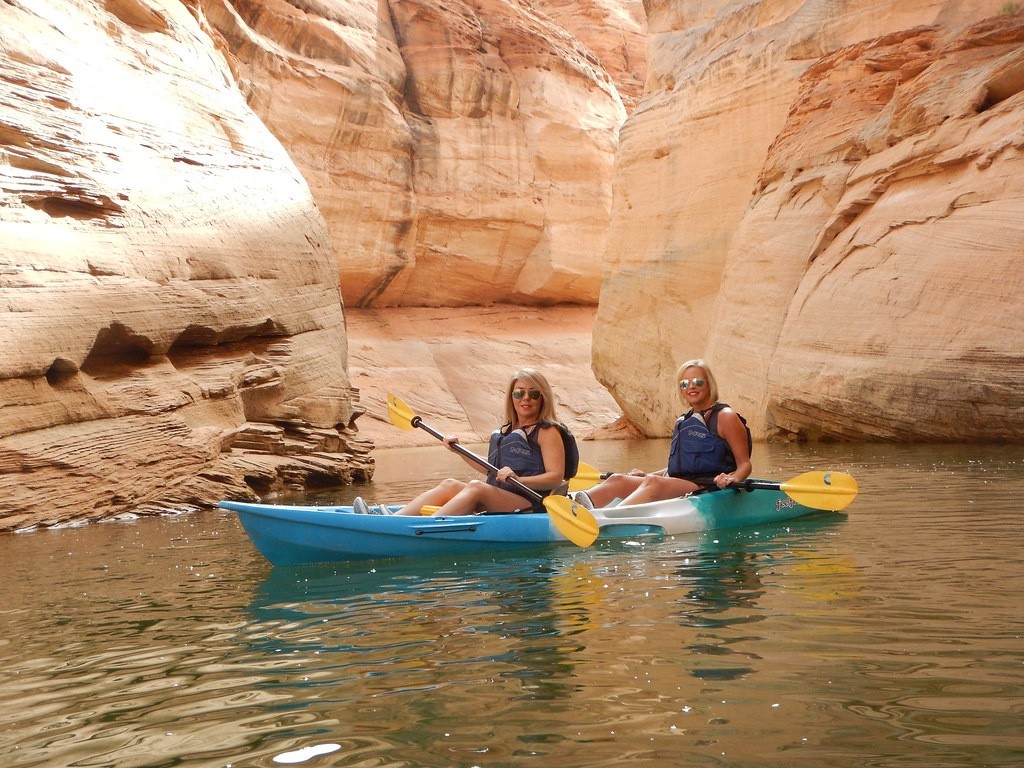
[217,479,820,568]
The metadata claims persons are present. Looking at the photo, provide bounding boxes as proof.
[353,369,580,516]
[576,359,752,510]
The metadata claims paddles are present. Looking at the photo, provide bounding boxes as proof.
[385,390,601,548]
[569,460,859,513]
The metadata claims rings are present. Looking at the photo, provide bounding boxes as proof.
[725,478,728,481]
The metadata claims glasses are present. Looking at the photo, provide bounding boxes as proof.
[678,378,707,390]
[510,388,542,400]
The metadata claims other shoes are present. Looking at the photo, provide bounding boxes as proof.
[379,503,394,515]
[353,496,382,515]
[574,490,595,511]
